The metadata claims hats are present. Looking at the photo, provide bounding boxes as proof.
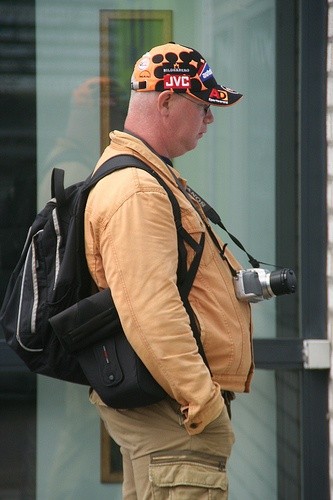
[130,42,244,107]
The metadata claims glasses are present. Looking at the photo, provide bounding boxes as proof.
[173,90,211,115]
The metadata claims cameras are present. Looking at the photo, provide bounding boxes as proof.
[233,268,297,303]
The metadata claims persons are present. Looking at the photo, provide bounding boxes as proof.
[86,43,257,500]
[43,77,126,211]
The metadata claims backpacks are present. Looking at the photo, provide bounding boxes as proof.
[0,154,198,410]
